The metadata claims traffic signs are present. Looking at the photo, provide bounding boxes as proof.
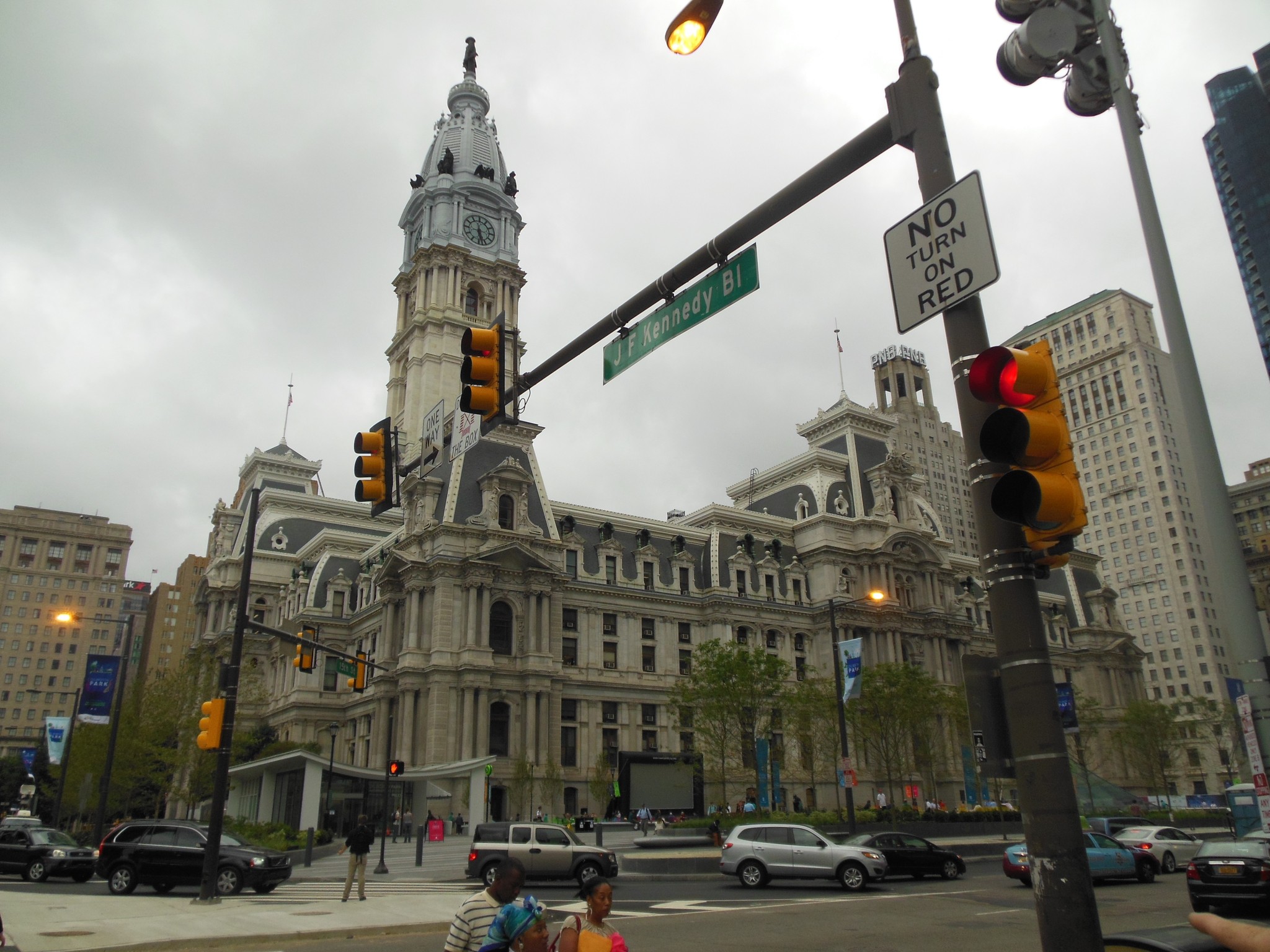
[419,398,445,480]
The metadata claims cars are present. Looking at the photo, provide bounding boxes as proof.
[1002,829,1163,888]
[831,830,966,881]
[0,810,42,827]
[1186,836,1270,919]
[1104,825,1204,874]
[1086,816,1158,837]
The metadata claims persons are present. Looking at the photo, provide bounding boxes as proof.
[505,788,1016,837]
[444,856,526,952]
[479,895,549,952]
[338,815,375,902]
[1188,911,1269,952]
[557,877,627,952]
[391,807,463,844]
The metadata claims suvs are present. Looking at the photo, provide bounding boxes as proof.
[95,817,293,897]
[464,821,618,887]
[718,822,892,893]
[0,824,100,883]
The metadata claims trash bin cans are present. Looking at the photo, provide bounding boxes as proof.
[575,817,585,832]
[585,817,594,832]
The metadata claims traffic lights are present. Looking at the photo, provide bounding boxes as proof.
[353,428,386,507]
[347,649,366,694]
[389,761,404,775]
[293,624,315,674]
[196,698,226,751]
[459,323,500,424]
[967,339,1091,571]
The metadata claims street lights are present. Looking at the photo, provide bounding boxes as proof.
[323,722,340,831]
[827,590,884,839]
[995,1,1269,748]
[54,613,135,850]
[23,688,80,821]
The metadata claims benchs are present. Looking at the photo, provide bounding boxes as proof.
[658,835,729,848]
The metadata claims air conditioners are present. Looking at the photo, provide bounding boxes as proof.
[796,644,804,649]
[610,580,618,585]
[770,597,776,602]
[797,675,805,681]
[606,662,695,751]
[683,590,691,596]
[567,622,573,627]
[681,634,689,639]
[349,746,354,752]
[644,630,653,635]
[604,625,611,630]
[741,592,748,597]
[797,601,804,606]
[647,585,655,590]
[768,641,776,646]
[739,638,746,643]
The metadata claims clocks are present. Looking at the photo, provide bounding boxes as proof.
[463,215,495,246]
[414,224,423,252]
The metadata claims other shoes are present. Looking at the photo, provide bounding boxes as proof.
[359,897,366,901]
[341,898,347,902]
